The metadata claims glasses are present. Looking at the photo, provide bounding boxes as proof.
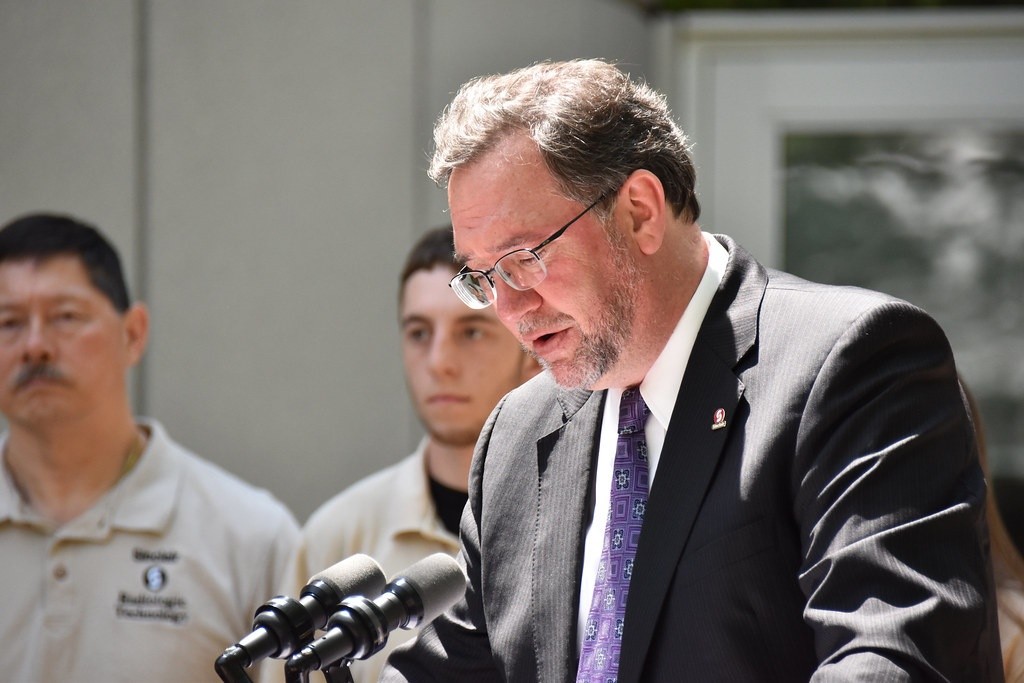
[447,188,612,310]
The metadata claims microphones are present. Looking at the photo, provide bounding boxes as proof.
[215,552,467,672]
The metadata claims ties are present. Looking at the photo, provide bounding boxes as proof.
[576,386,651,683]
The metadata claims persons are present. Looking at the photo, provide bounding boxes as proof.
[1,210,298,683]
[380,58,1006,683]
[262,227,545,682]
